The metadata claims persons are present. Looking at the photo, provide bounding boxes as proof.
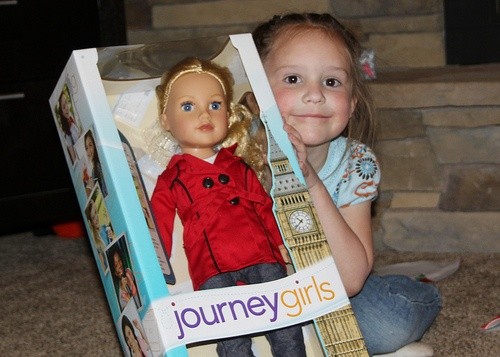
[110,251,141,310]
[80,128,108,200]
[59,90,85,165]
[146,57,310,357]
[249,11,441,356]
[87,202,121,274]
[118,314,154,356]
